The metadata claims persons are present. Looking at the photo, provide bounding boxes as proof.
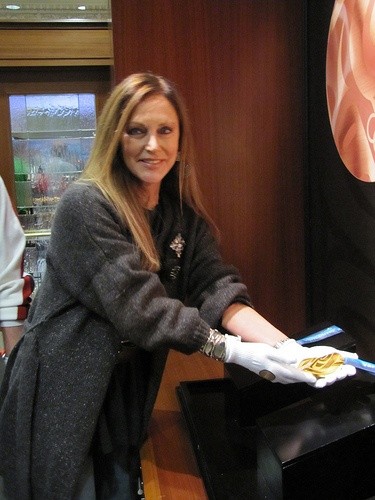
[26,138,79,197]
[0,72,358,500]
[0,175,36,382]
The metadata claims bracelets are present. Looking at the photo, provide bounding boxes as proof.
[272,337,290,349]
[204,329,226,362]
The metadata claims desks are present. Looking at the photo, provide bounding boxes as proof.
[140,348,224,500]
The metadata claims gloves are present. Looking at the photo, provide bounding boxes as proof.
[276,339,359,388]
[224,334,316,384]
